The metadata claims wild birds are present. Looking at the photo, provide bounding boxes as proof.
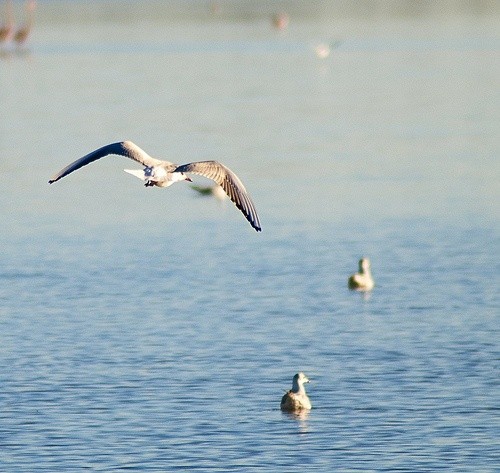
[279,372,313,411]
[348,256,374,291]
[47,140,262,232]
